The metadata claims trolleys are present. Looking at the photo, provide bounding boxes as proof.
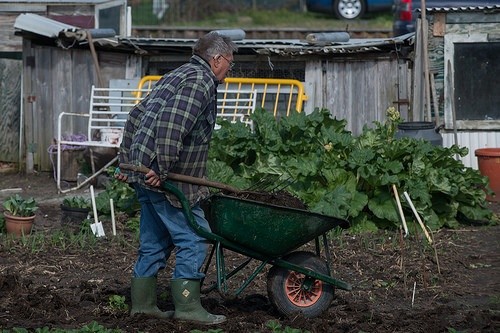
[127,173,354,321]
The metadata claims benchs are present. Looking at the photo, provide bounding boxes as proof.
[56,75,309,193]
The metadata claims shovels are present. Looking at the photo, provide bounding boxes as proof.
[89,185,106,238]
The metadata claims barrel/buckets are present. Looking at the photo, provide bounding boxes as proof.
[475,148,500,202]
[393,121,446,148]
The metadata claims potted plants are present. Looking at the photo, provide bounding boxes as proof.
[62,197,92,219]
[3,194,36,236]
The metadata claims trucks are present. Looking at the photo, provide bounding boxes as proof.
[305,0,500,23]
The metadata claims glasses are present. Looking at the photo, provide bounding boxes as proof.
[213,53,235,69]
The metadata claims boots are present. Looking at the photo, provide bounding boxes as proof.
[130,276,175,319]
[170,278,227,324]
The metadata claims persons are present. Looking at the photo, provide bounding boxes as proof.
[114,33,238,324]
